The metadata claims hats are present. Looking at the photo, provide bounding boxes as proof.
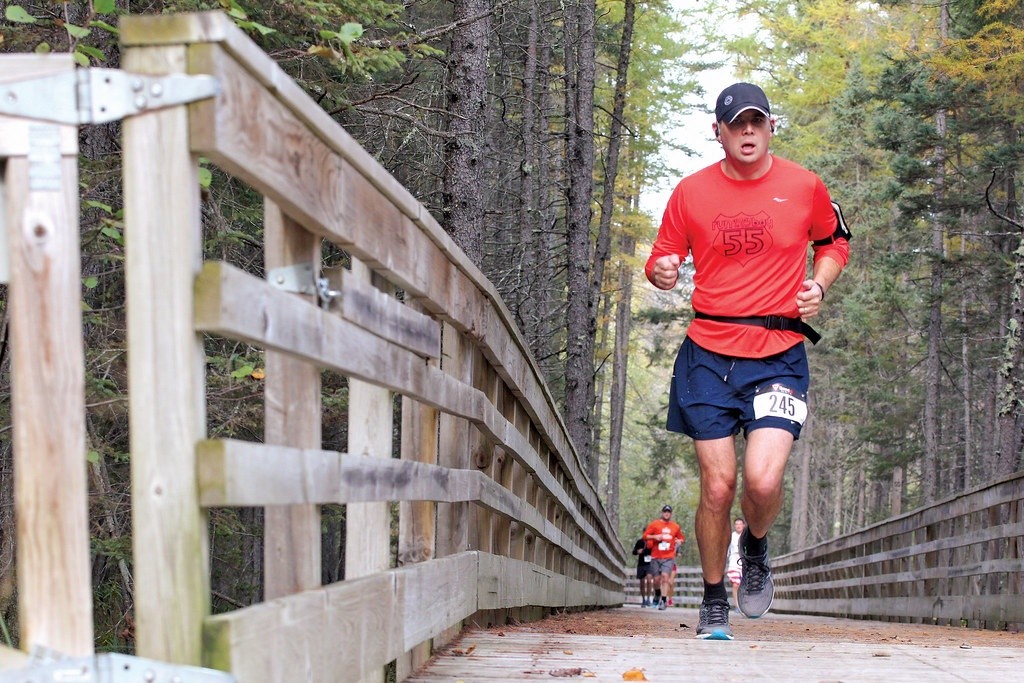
[662,506,671,512]
[715,83,770,125]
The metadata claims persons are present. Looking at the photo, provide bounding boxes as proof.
[644,83,850,638]
[726,518,745,613]
[632,504,685,610]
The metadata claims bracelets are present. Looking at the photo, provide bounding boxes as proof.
[815,282,824,301]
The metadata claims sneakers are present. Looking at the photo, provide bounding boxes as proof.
[646,599,651,606]
[653,595,661,608]
[641,602,646,608]
[659,600,667,610]
[667,598,672,606]
[696,599,734,640]
[737,526,775,618]
